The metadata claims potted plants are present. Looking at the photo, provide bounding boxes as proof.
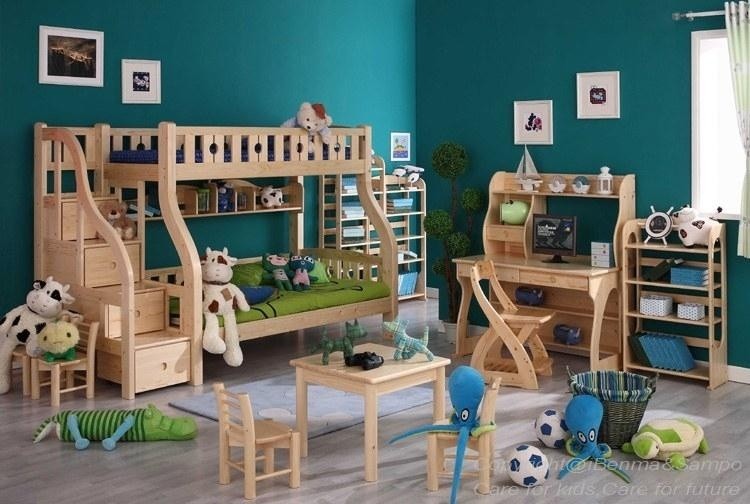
[424,139,486,345]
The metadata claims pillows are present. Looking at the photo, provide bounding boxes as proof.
[310,259,331,284]
[230,262,265,289]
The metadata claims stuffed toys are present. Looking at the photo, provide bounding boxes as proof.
[392,164,425,190]
[279,101,333,153]
[622,419,710,471]
[556,395,633,484]
[500,200,529,225]
[0,275,76,394]
[262,251,293,291]
[260,185,283,207]
[310,312,433,370]
[36,314,80,362]
[96,200,136,240]
[200,246,251,368]
[671,202,722,249]
[516,285,543,306]
[32,404,198,451]
[554,323,582,346]
[383,365,497,504]
[290,252,319,293]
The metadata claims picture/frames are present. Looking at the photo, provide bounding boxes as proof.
[390,131,410,162]
[514,100,553,145]
[39,25,104,88]
[121,58,161,104]
[576,72,621,119]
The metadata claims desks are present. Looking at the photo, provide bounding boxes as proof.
[290,343,451,484]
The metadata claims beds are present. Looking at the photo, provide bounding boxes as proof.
[33,120,398,398]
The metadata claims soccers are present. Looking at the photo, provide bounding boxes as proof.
[506,445,548,487]
[533,407,571,450]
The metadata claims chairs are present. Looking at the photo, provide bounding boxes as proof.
[471,258,555,389]
[31,316,99,398]
[11,310,69,396]
[427,375,501,495]
[212,382,300,500]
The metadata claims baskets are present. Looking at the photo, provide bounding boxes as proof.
[566,364,659,449]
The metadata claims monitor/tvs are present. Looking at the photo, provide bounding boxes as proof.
[532,214,577,263]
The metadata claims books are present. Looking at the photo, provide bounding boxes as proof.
[627,331,697,373]
[341,184,358,195]
[671,265,709,287]
[397,269,418,296]
[386,197,414,214]
[342,206,365,219]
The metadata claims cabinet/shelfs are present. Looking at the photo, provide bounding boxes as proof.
[460,170,636,372]
[319,155,428,301]
[622,217,729,390]
